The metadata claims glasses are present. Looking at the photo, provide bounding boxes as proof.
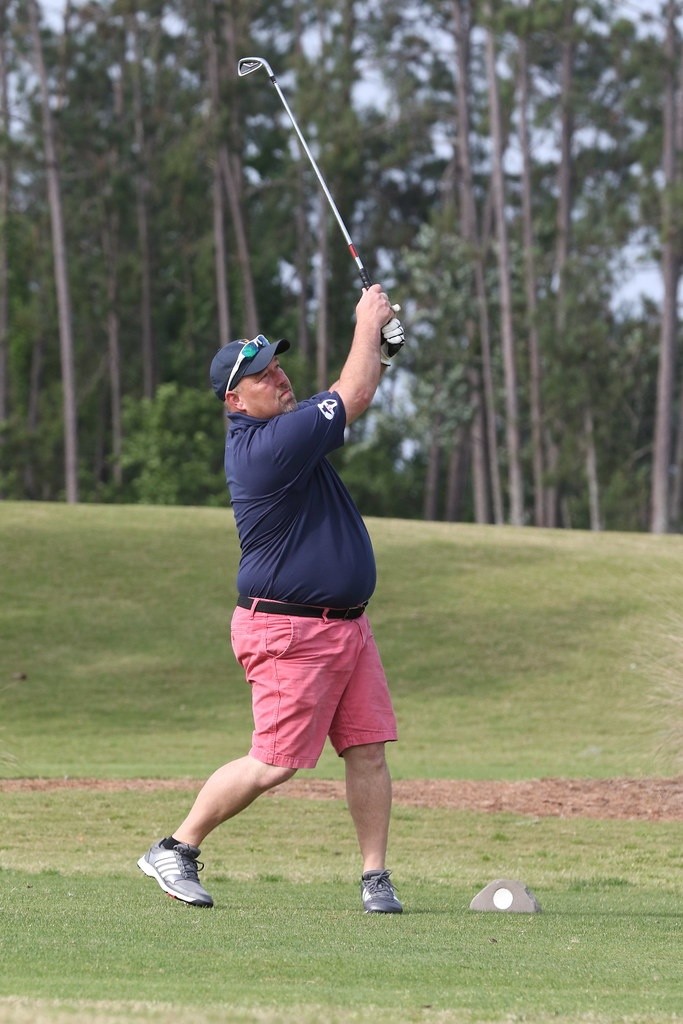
[225,334,271,398]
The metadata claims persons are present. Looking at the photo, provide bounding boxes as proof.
[135,283,406,914]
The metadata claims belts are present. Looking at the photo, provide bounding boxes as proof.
[236,595,369,620]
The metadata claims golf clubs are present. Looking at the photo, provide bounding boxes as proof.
[236,54,371,286]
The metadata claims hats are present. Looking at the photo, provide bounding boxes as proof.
[210,337,290,402]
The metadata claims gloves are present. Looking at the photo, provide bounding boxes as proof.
[376,304,405,366]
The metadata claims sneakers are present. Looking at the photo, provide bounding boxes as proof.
[137,838,214,908]
[359,868,402,914]
